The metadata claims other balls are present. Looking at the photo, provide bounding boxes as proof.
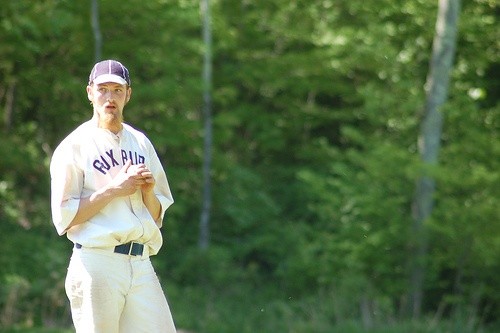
[127,165,145,179]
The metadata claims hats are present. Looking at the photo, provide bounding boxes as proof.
[89,59,131,87]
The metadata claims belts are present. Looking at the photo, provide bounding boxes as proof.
[76,243,144,256]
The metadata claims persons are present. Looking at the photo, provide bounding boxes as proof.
[49,59,178,333]
